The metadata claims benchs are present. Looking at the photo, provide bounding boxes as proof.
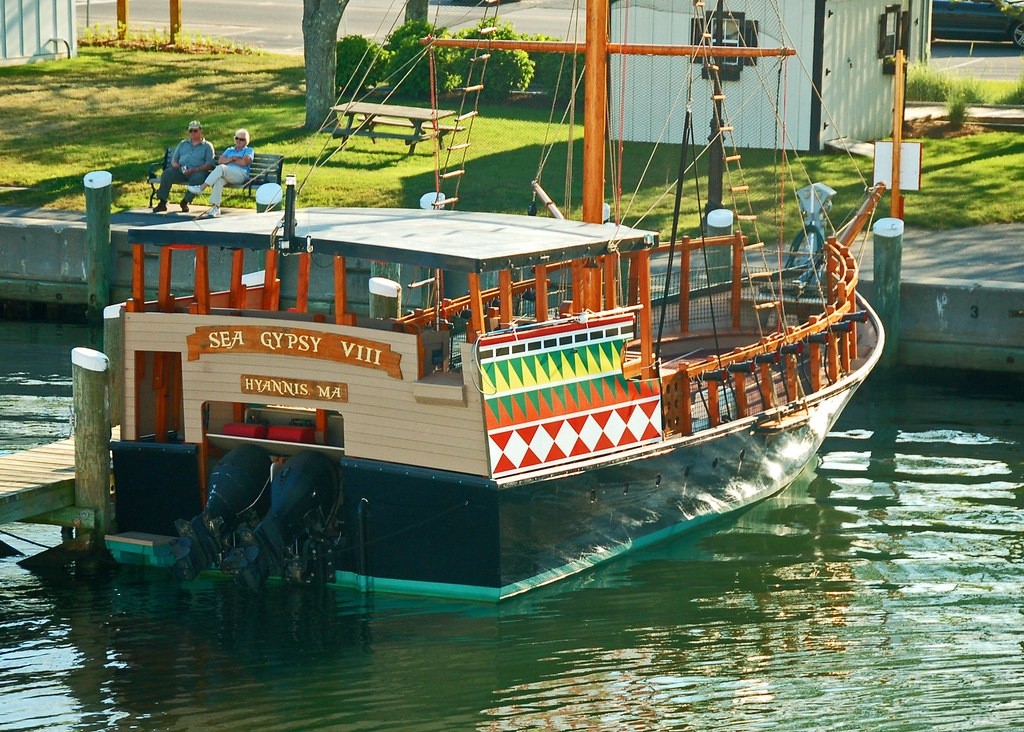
[146,147,283,208]
[320,116,466,145]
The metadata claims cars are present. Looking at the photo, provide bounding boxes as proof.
[930,1,1024,50]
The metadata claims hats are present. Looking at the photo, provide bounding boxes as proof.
[189,120,201,128]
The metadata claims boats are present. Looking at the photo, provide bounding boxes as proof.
[103,0,885,606]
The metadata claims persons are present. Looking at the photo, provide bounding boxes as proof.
[186,129,254,217]
[152,121,215,212]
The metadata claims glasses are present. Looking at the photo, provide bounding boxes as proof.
[189,129,200,133]
[235,137,246,141]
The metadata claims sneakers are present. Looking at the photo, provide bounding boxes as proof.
[208,206,221,217]
[152,202,167,212]
[180,200,189,212]
[188,185,203,195]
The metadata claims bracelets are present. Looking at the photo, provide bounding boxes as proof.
[232,156,234,161]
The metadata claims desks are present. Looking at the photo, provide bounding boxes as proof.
[329,102,456,153]
